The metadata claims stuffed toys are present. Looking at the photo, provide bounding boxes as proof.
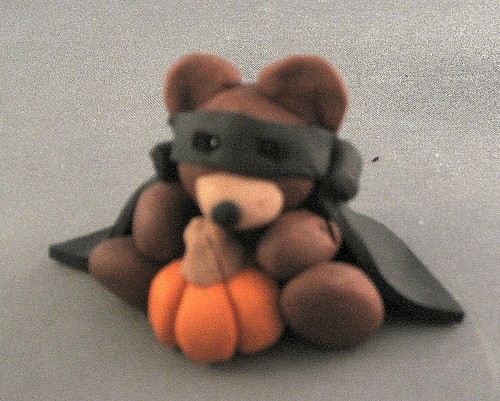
[48,52,464,367]
